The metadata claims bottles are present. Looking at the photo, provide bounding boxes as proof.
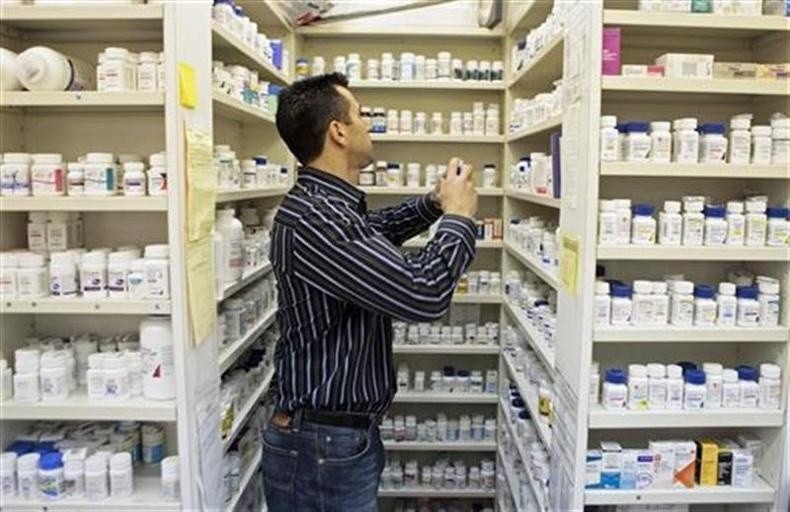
[601,111,789,163]
[0,243,172,300]
[1,423,167,506]
[589,432,768,490]
[0,40,166,89]
[296,50,505,82]
[603,194,790,246]
[359,100,501,136]
[210,3,285,73]
[594,358,781,409]
[382,413,494,441]
[210,142,289,189]
[384,450,495,489]
[595,263,782,328]
[377,495,495,511]
[391,316,500,346]
[156,453,180,506]
[397,361,497,394]
[0,147,166,195]
[220,322,284,442]
[1,315,172,401]
[358,159,496,189]
[497,6,566,512]
[220,390,283,511]
[216,204,278,285]
[25,211,85,255]
[476,217,504,239]
[212,52,279,119]
[218,271,278,351]
[454,271,502,294]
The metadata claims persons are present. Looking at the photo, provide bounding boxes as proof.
[261,71,477,512]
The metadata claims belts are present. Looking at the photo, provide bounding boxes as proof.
[274,405,373,432]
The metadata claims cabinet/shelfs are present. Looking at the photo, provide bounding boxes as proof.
[1,1,790,512]
